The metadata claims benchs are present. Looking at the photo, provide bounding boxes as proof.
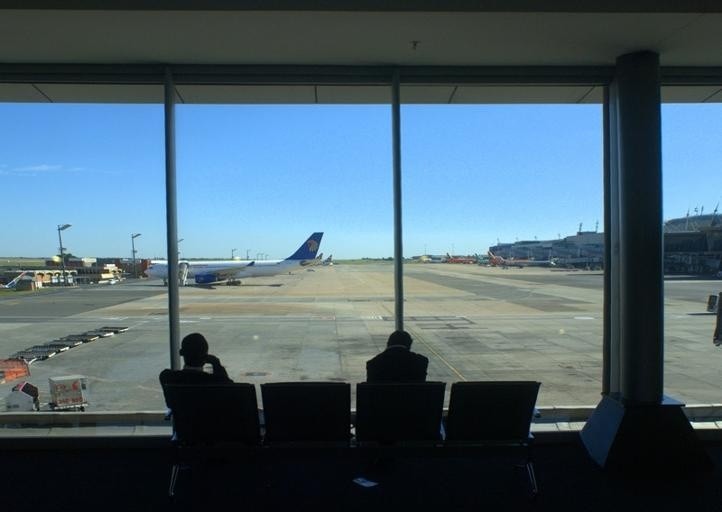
[163,381,540,496]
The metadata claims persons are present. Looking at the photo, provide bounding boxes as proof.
[367,331,428,384]
[160,334,232,409]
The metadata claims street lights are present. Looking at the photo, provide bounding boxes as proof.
[231,248,270,261]
[57,223,72,287]
[131,232,143,279]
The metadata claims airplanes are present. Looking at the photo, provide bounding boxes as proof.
[137,233,334,286]
[445,252,534,270]
